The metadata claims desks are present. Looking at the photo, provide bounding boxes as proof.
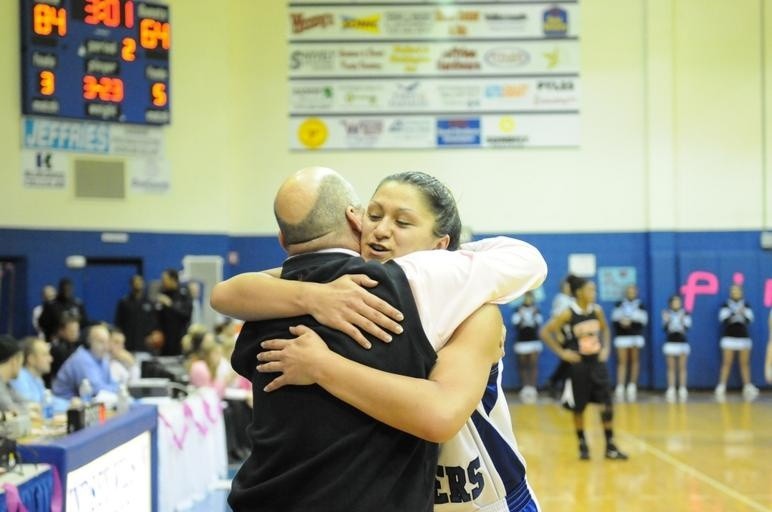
[0,383,235,510]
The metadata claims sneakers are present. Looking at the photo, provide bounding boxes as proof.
[605,449,627,459]
[665,386,689,398]
[713,383,727,396]
[580,452,590,459]
[614,383,638,396]
[519,385,538,396]
[743,383,760,396]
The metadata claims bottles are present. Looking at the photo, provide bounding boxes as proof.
[117,383,129,414]
[79,377,93,405]
[43,389,54,426]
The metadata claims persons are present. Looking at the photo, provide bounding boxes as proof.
[661,296,691,398]
[511,290,543,403]
[538,277,628,461]
[2,271,254,462]
[713,285,760,399]
[545,276,573,399]
[611,284,648,401]
